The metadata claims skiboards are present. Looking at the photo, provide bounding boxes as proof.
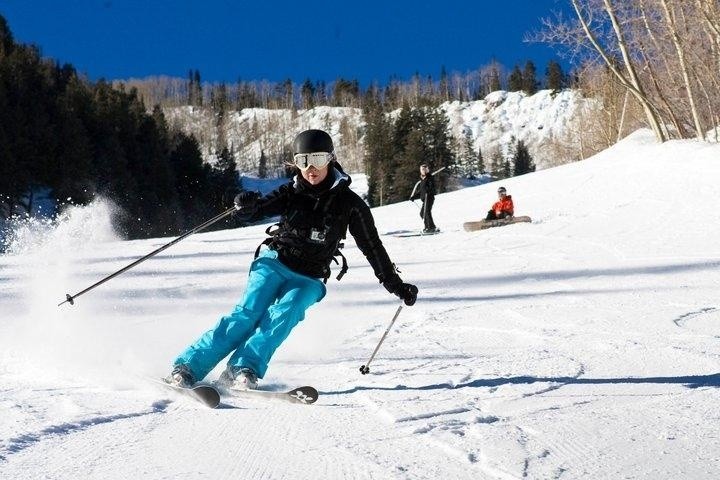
[382,229,442,238]
[151,374,319,407]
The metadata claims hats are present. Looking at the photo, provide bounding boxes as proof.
[498,187,506,194]
[420,164,429,175]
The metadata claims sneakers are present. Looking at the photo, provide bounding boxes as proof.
[226,366,258,389]
[168,363,193,388]
[420,228,435,233]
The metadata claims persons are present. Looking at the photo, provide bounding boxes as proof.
[408,164,436,233]
[485,186,514,218]
[160,129,418,390]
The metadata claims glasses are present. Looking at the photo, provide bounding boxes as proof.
[293,149,335,172]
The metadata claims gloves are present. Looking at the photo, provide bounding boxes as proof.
[394,283,418,305]
[234,191,258,214]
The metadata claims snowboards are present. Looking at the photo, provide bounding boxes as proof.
[463,216,532,232]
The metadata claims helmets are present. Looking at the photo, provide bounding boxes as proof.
[293,130,334,157]
